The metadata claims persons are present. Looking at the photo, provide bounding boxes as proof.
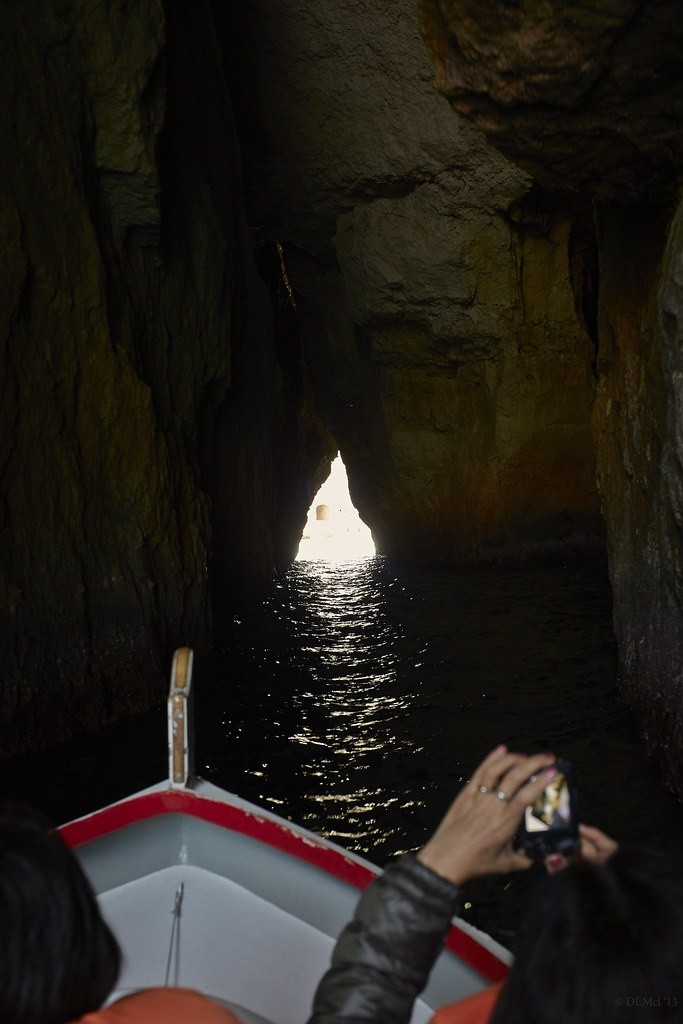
[301,746,683,1023]
[0,816,263,1024]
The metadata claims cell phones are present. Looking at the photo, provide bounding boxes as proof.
[510,757,581,877]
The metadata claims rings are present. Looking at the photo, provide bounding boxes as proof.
[477,785,491,794]
[496,791,511,803]
